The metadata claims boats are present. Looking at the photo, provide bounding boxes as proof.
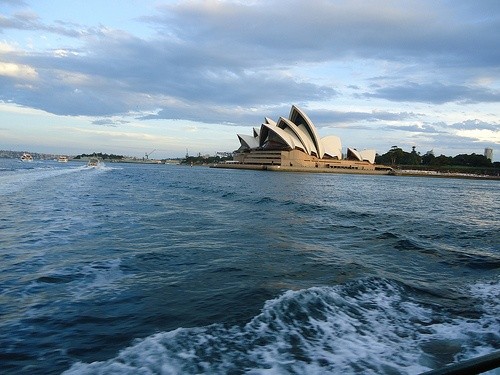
[57,156,67,163]
[21,153,33,162]
[86,158,100,168]
